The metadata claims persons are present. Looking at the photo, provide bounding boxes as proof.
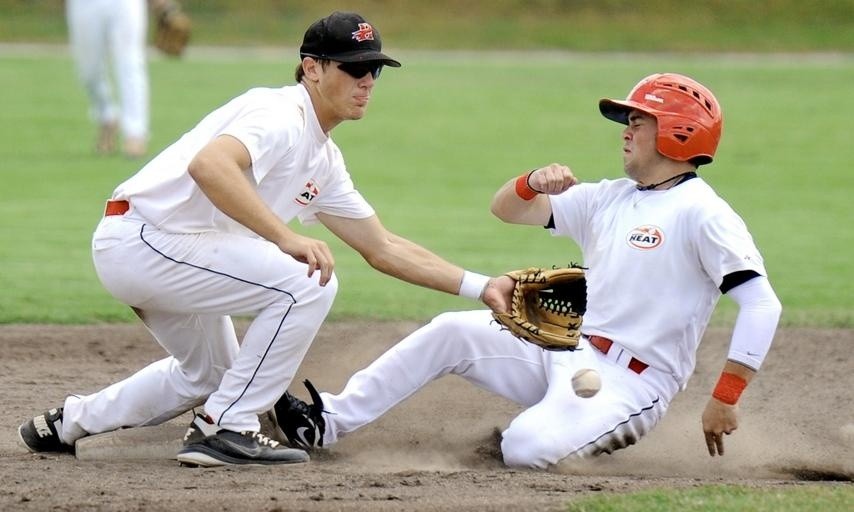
[16,10,546,468]
[267,72,783,471]
[65,1,191,157]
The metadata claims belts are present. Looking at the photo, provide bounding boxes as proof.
[585,336,649,375]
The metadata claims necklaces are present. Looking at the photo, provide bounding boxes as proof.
[636,171,697,190]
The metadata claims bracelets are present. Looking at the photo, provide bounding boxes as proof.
[458,270,491,300]
[515,168,545,201]
[711,371,747,405]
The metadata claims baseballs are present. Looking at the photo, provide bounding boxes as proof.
[573,368,601,397]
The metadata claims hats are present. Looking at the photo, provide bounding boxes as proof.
[298,10,402,69]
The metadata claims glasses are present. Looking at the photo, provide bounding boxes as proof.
[336,59,384,80]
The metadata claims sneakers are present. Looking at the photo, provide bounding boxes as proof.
[269,379,326,453]
[17,404,77,459]
[176,411,310,470]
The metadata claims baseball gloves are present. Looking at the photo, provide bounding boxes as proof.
[157,4,191,55]
[492,264,586,352]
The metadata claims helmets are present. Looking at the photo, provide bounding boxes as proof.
[598,71,725,165]
